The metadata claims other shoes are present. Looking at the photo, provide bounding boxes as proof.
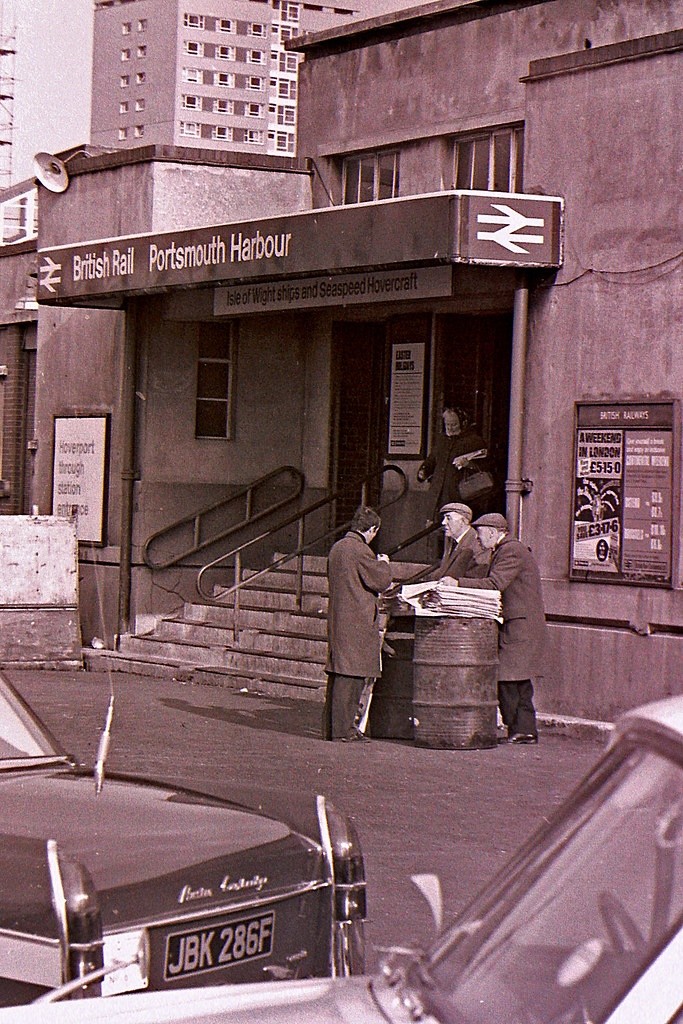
[333,728,370,743]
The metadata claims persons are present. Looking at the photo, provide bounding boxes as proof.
[416,405,492,564]
[320,505,393,744]
[436,503,547,745]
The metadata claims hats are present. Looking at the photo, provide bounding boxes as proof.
[469,513,507,528]
[439,503,472,521]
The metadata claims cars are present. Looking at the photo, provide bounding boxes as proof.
[1,698,683,1024]
[0,655,371,1008]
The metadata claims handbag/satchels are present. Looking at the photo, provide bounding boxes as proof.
[459,463,494,500]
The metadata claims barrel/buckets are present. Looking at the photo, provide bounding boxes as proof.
[368,615,414,738]
[413,615,500,749]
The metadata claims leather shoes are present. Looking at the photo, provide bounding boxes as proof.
[496,732,536,744]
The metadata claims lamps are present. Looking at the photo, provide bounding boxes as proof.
[33,151,93,193]
